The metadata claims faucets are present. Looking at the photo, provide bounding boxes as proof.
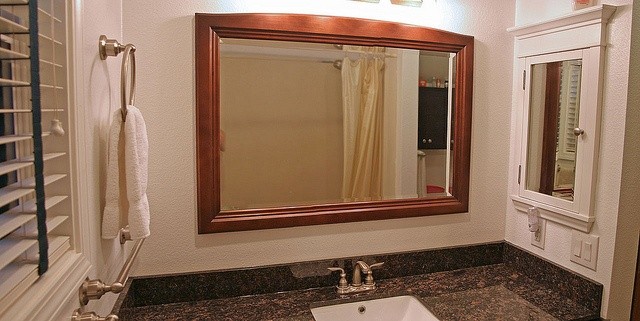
[351,260,371,286]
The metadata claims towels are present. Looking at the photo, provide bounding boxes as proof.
[101,109,152,242]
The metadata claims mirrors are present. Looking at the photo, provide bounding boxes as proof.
[506,4,617,235]
[196,13,476,235]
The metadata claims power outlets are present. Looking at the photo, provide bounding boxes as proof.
[528,217,547,250]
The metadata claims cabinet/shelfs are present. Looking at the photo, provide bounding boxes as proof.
[418,86,454,150]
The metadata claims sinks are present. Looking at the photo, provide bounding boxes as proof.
[310,295,440,321]
